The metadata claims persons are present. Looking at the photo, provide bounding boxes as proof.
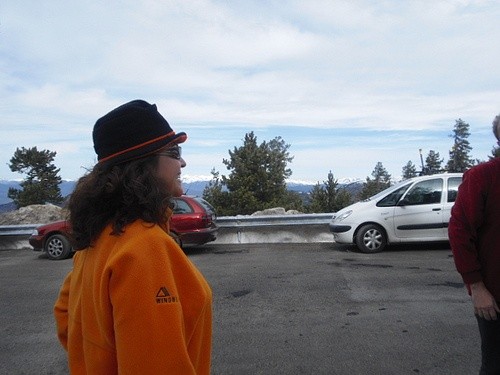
[446,114,500,375]
[53,98,214,375]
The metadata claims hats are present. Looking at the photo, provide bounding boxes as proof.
[93,100,187,170]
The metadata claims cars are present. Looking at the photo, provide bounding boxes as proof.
[329,173,465,253]
[29,194,218,260]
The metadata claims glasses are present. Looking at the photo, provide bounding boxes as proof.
[141,146,182,160]
